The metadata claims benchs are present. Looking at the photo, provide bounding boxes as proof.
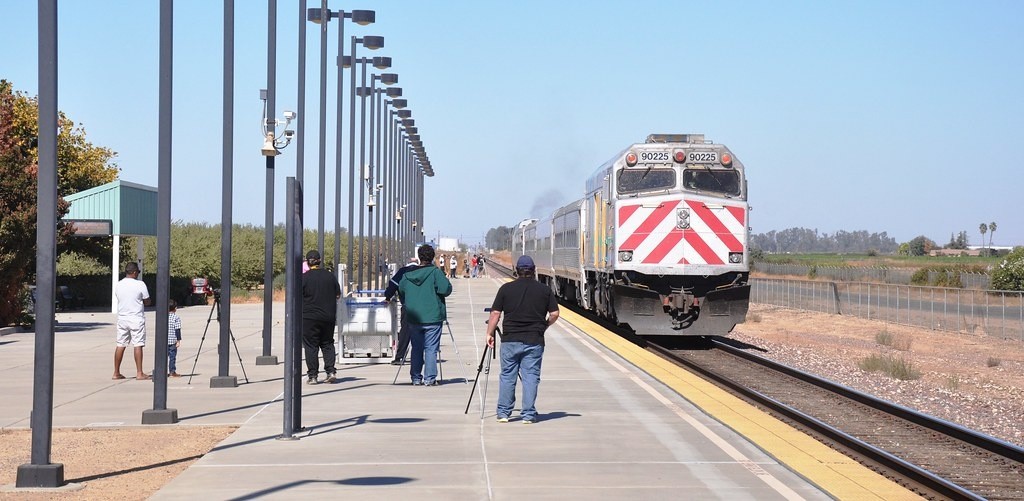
[29,286,60,314]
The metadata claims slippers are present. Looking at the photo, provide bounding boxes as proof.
[112,374,126,380]
[136,373,149,380]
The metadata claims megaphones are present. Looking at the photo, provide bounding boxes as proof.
[367,195,376,206]
[396,211,401,219]
[260,133,276,156]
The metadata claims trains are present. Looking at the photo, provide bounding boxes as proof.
[511,132,751,336]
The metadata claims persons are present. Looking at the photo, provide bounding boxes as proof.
[432,253,486,279]
[168,299,182,377]
[399,244,453,386]
[112,262,151,380]
[301,253,418,385]
[486,255,559,424]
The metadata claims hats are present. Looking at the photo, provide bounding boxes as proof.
[306,250,320,261]
[516,255,534,268]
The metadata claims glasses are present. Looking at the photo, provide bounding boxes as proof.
[135,269,140,273]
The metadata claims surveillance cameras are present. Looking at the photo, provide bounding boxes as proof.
[284,129,294,139]
[377,183,383,189]
[283,111,296,120]
[402,205,407,208]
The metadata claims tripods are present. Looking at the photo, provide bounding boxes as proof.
[393,315,468,385]
[465,308,523,420]
[188,290,248,383]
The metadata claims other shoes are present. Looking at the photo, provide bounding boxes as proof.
[391,358,404,365]
[497,417,508,422]
[324,373,335,383]
[413,383,420,386]
[169,373,181,378]
[306,378,317,384]
[522,420,534,423]
[427,382,438,386]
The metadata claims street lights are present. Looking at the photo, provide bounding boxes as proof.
[308,8,375,266]
[369,73,398,297]
[337,56,392,296]
[351,35,384,296]
[357,86,434,296]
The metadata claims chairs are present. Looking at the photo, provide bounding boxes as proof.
[60,286,85,310]
